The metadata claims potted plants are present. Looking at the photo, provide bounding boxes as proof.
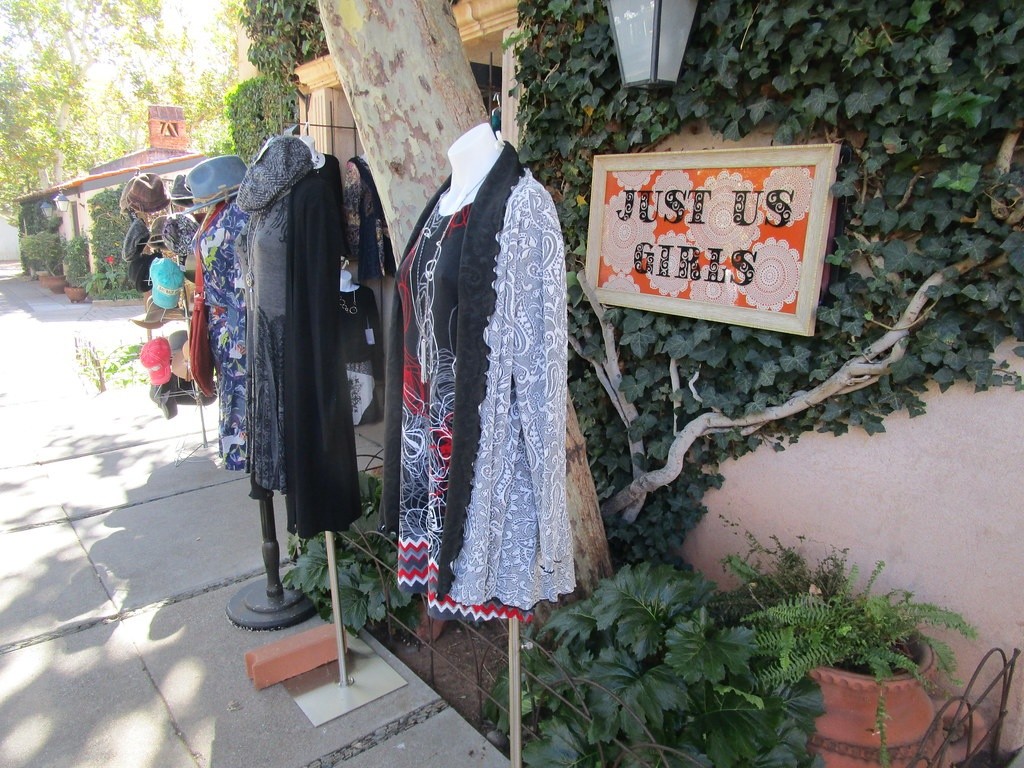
[20,231,66,294]
[707,513,978,768]
[63,235,89,304]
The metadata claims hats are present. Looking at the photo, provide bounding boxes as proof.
[139,336,172,385]
[148,216,174,243]
[119,172,171,215]
[161,216,198,257]
[168,330,188,355]
[121,217,151,262]
[148,256,185,310]
[170,174,195,200]
[235,134,316,217]
[127,252,163,293]
[128,294,194,329]
[339,269,360,292]
[170,339,204,382]
[179,155,253,216]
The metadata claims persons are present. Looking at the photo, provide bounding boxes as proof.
[338,267,381,425]
[380,123,575,623]
[345,156,397,278]
[186,155,253,472]
[235,136,362,541]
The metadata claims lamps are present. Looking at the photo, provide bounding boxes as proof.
[40,200,55,221]
[52,187,70,213]
[606,0,699,90]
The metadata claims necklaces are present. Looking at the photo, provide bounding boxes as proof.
[340,290,358,315]
[410,174,488,384]
[246,213,261,286]
